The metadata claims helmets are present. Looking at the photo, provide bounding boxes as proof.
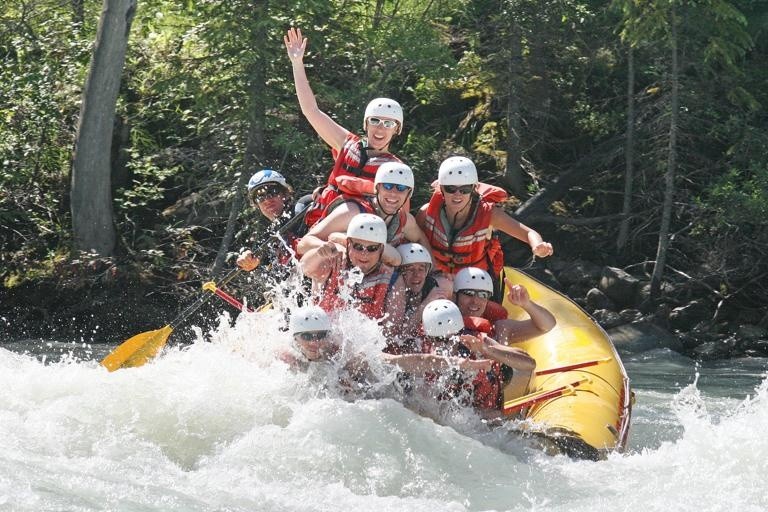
[288,307,330,333]
[374,161,414,197]
[363,97,404,135]
[397,243,433,276]
[438,156,478,186]
[453,267,493,299]
[248,170,288,198]
[422,299,465,337]
[347,213,387,246]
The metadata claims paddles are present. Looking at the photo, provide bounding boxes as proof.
[99,206,308,373]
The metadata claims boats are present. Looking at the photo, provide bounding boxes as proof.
[250,265,635,459]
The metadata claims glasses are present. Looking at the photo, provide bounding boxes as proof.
[350,238,382,251]
[384,183,408,191]
[445,185,475,195]
[367,116,398,129]
[252,184,280,202]
[301,330,327,341]
[462,289,492,299]
